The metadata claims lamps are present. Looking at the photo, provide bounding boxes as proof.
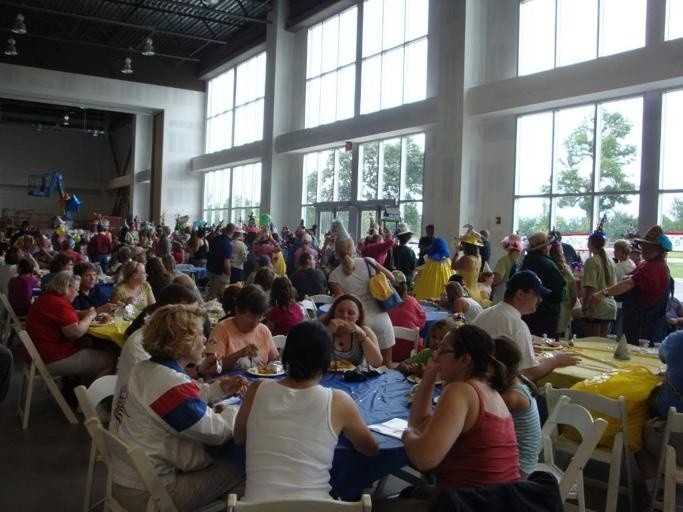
[3,0,156,74]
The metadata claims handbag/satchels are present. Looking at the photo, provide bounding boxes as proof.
[365,257,403,312]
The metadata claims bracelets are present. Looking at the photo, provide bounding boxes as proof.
[360,335,368,343]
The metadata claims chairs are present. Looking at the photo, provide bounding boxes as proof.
[0,264,683,511]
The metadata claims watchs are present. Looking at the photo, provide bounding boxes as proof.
[603,288,609,298]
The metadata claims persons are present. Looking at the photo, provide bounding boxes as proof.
[0,206,682,510]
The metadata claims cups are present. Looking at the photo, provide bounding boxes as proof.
[655,342,662,356]
[607,334,617,350]
[639,339,651,354]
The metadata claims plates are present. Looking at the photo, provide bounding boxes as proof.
[328,360,357,372]
[433,395,440,403]
[246,365,285,377]
[536,340,565,349]
[408,375,442,384]
[90,317,116,327]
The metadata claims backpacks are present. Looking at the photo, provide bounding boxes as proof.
[583,295,619,322]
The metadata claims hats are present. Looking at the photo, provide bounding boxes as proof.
[634,225,673,248]
[392,269,407,284]
[396,222,414,237]
[456,229,485,247]
[232,223,246,234]
[526,231,555,250]
[500,233,530,252]
[507,270,552,294]
[300,233,313,243]
